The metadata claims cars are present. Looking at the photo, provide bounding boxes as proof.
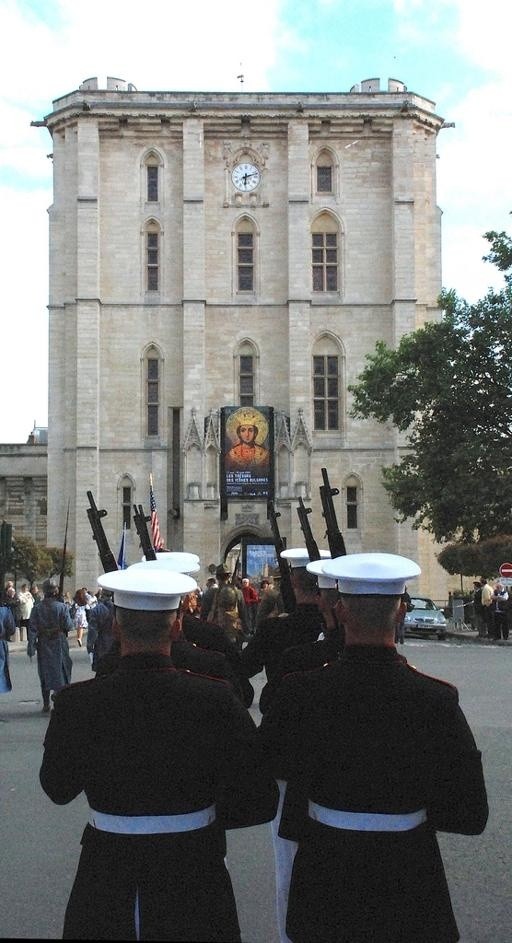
[401,593,447,640]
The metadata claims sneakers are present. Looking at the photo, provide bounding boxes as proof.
[477,632,510,641]
[43,705,51,712]
[77,638,83,647]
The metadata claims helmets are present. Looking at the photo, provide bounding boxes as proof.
[217,584,238,609]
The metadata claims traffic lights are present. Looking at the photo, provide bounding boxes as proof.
[2,523,16,557]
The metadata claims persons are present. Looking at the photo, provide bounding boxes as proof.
[226,408,270,467]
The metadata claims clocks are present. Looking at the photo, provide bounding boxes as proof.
[230,159,263,193]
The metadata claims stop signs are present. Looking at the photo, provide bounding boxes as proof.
[498,562,512,579]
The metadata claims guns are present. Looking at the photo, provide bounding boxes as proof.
[267,502,296,612]
[87,491,117,573]
[297,496,321,562]
[132,504,157,561]
[319,468,347,559]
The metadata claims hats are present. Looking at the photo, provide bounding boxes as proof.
[212,563,232,575]
[305,558,337,590]
[321,553,423,596]
[96,551,201,613]
[42,578,57,593]
[279,548,332,568]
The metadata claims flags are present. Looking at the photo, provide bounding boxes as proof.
[150,477,164,551]
[118,527,128,570]
